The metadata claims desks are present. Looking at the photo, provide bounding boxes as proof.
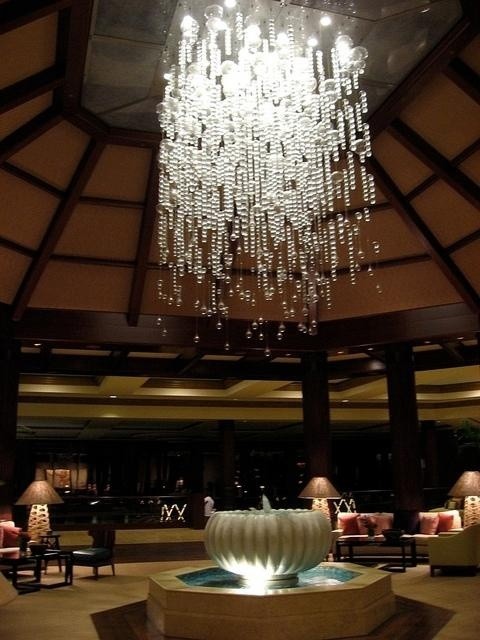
[39,535,62,575]
[428,507,463,525]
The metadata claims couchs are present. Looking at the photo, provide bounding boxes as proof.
[331,509,463,556]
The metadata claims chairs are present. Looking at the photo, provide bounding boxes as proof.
[0,520,23,558]
[427,524,480,577]
[71,525,115,580]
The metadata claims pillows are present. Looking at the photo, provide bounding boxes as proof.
[3,526,22,548]
[339,513,454,535]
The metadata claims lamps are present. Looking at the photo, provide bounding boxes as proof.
[157,0,383,358]
[447,471,480,527]
[15,481,64,541]
[298,477,341,521]
